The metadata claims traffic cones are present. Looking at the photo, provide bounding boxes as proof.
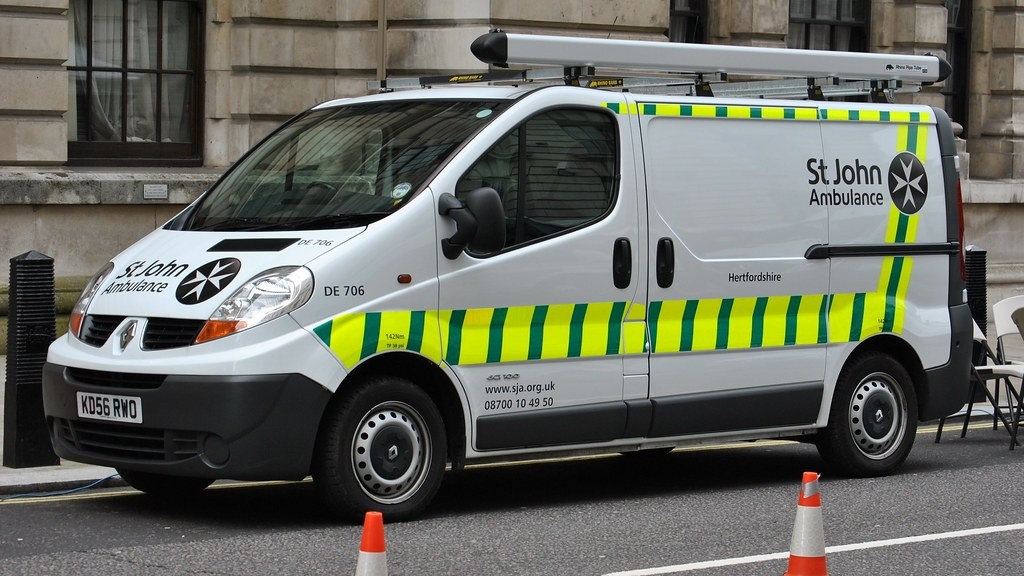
[783,472,831,576]
[353,511,389,576]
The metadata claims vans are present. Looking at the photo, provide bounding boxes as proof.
[41,24,974,525]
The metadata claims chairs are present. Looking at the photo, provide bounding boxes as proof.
[934,295,1024,452]
